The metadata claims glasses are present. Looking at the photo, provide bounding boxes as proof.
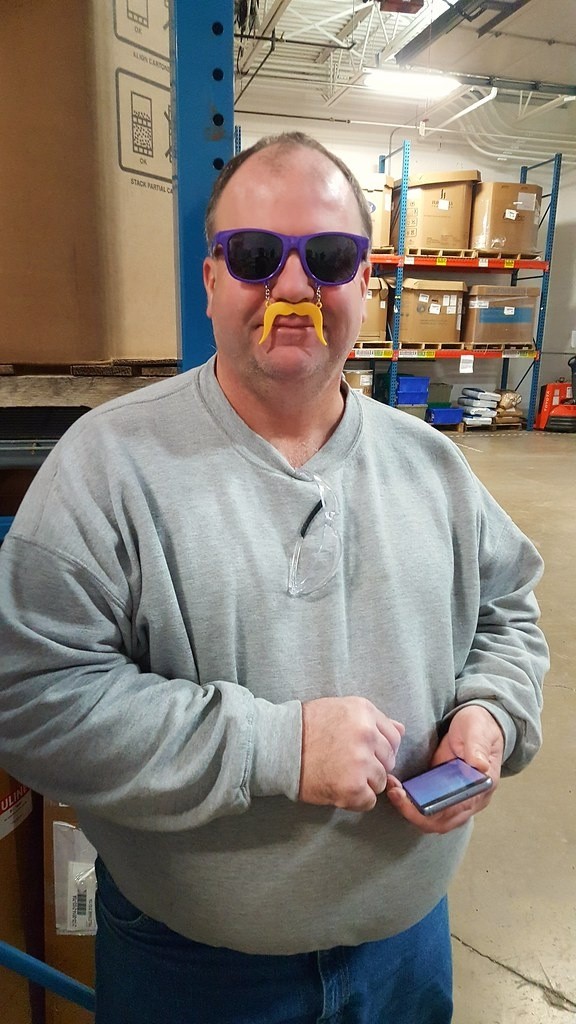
[209,228,369,287]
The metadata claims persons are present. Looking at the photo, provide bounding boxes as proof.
[0,132,552,1023]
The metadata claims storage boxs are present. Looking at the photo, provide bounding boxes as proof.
[354,276,391,341]
[379,373,464,425]
[382,276,468,342]
[464,285,541,343]
[0,0,183,362]
[390,169,482,249]
[470,170,542,252]
[356,174,394,247]
[0,768,98,1024]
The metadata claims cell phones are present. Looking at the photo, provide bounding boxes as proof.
[401,756,493,815]
